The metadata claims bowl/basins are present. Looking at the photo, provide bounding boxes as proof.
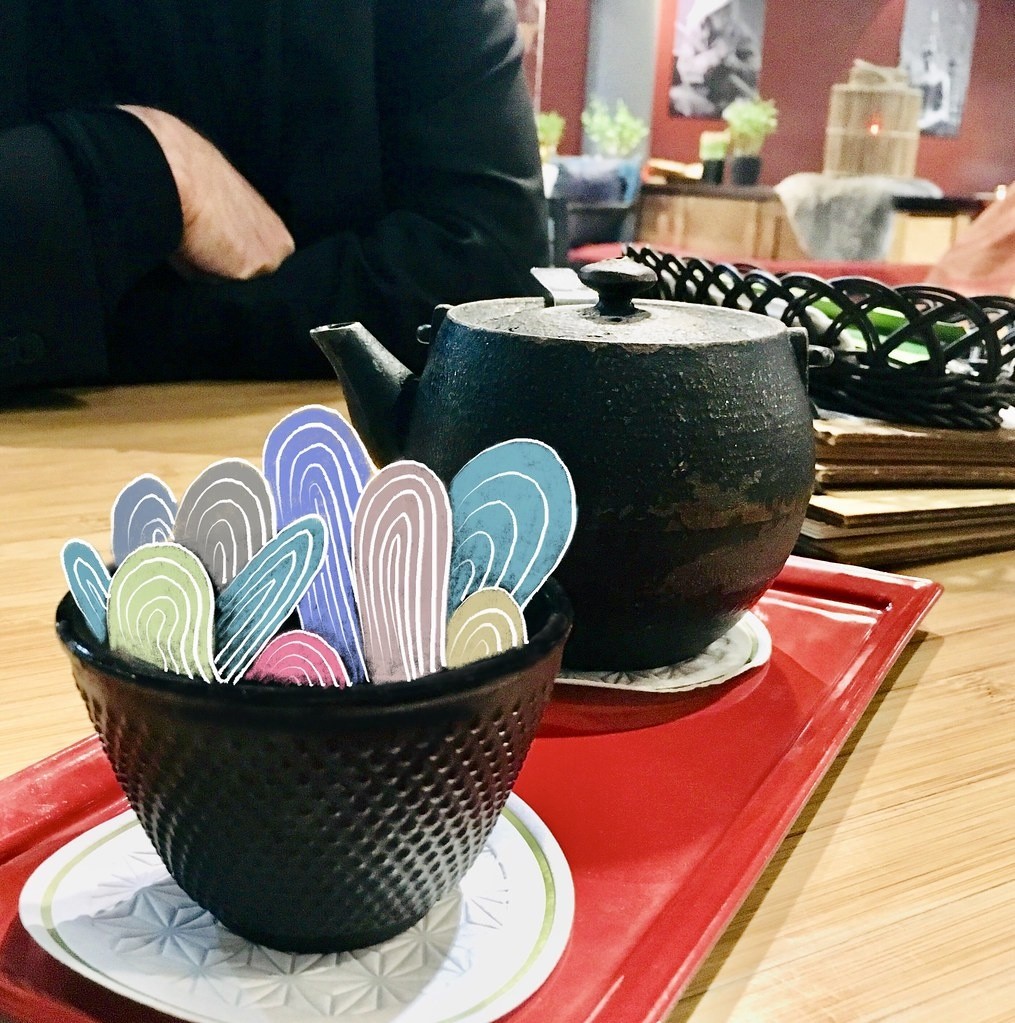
[56,568,574,954]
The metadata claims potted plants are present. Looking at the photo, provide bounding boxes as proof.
[699,130,727,184]
[531,112,564,162]
[721,99,778,185]
[578,95,652,159]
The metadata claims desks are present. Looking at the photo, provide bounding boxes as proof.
[1,374,1012,1023]
[632,182,973,266]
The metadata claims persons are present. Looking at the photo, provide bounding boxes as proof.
[0,0,555,391]
[667,0,762,119]
[912,44,950,137]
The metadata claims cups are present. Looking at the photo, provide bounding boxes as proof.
[730,158,761,185]
[703,161,725,184]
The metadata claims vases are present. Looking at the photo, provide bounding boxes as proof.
[565,203,631,248]
[56,578,570,951]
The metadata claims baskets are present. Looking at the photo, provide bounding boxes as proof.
[616,243,1014,429]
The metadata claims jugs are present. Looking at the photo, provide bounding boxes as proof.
[310,261,816,674]
